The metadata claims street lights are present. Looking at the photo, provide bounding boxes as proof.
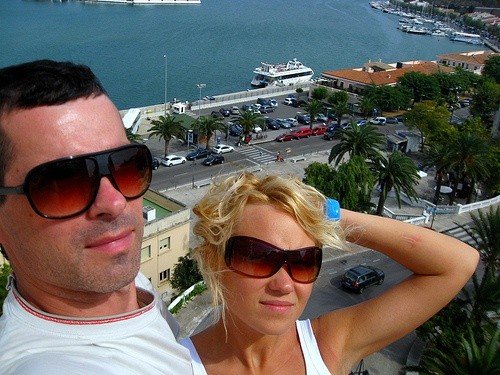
[196,83,207,150]
[163,54,167,118]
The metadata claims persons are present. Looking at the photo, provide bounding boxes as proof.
[0,59,193,375]
[191,172,480,375]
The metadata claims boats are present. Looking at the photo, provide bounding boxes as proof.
[368,0,486,47]
[250,58,315,88]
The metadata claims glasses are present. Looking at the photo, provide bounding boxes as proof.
[224,236,323,284]
[0,143,152,219]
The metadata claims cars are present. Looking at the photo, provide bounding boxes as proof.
[211,144,235,155]
[340,264,385,294]
[186,95,472,162]
[201,154,224,168]
[161,154,187,167]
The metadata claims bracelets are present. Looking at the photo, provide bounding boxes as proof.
[323,196,340,222]
